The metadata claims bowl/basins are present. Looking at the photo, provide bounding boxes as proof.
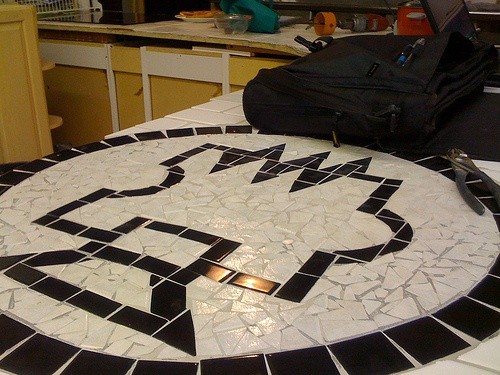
[213,13,253,35]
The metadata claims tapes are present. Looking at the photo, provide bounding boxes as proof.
[314,12,335,35]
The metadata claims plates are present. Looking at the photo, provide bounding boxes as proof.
[174,14,214,22]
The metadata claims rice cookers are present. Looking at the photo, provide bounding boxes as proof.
[397,0,433,35]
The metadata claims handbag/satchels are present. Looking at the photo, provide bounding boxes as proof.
[240,30,500,143]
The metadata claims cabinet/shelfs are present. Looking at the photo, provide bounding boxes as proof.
[0,0,302,164]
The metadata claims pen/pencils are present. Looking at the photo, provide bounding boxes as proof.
[397,45,412,65]
